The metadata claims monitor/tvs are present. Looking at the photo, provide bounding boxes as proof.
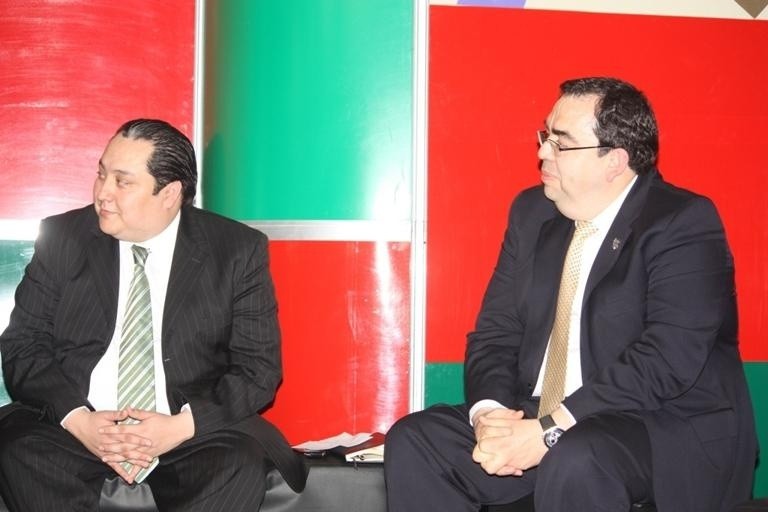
[332,431,384,463]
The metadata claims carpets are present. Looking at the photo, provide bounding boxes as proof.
[536,219,600,419]
[116,243,160,484]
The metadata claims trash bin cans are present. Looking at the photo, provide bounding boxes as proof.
[539,415,566,448]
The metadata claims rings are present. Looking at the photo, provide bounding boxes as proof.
[302,452,327,457]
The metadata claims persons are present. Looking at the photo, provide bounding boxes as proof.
[383,77,757,512]
[0,119,281,512]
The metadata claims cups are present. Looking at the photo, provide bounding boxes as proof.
[537,130,612,153]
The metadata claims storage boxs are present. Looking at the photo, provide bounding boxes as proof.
[98,435,768,511]
